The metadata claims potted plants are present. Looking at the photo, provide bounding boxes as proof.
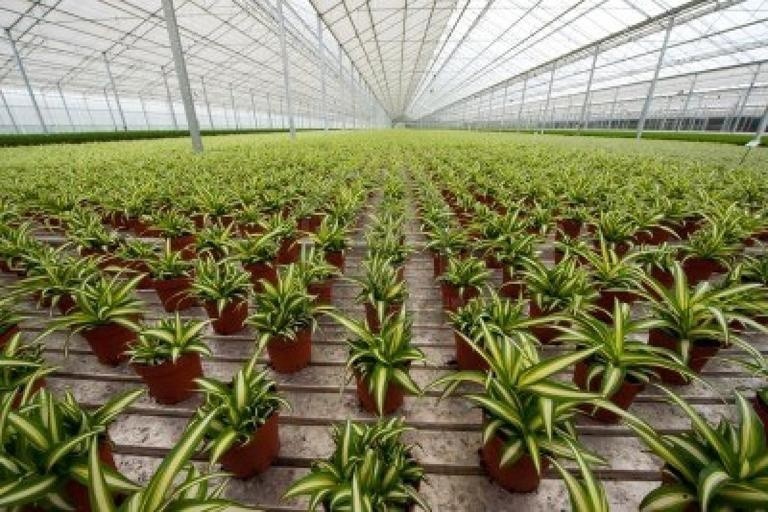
[1,164,767,512]
